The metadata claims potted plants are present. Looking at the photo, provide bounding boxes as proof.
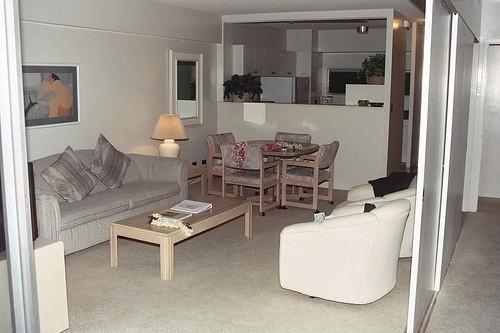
[222,72,264,102]
[360,54,385,84]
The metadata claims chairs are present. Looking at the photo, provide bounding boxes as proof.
[282,141,339,212]
[274,132,312,194]
[206,132,239,198]
[220,145,281,216]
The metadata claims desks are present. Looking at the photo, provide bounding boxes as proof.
[243,140,319,210]
[0,236,70,333]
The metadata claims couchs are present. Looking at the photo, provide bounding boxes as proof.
[33,149,189,255]
[348,175,416,258]
[278,198,410,305]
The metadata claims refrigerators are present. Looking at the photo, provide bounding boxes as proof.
[260,76,309,103]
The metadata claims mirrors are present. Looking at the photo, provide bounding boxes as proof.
[169,49,204,129]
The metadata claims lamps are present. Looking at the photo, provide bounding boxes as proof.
[151,114,189,157]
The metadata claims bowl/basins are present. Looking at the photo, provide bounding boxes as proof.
[358,100,369,106]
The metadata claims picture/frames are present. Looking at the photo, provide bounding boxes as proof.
[22,63,81,129]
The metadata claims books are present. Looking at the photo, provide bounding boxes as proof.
[160,199,213,220]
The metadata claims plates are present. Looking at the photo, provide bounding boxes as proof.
[368,102,384,107]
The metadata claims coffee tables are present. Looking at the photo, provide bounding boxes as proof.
[108,194,253,279]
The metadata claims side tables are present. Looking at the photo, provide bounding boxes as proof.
[188,164,206,195]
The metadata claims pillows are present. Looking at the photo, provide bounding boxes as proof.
[91,133,131,190]
[40,145,100,202]
